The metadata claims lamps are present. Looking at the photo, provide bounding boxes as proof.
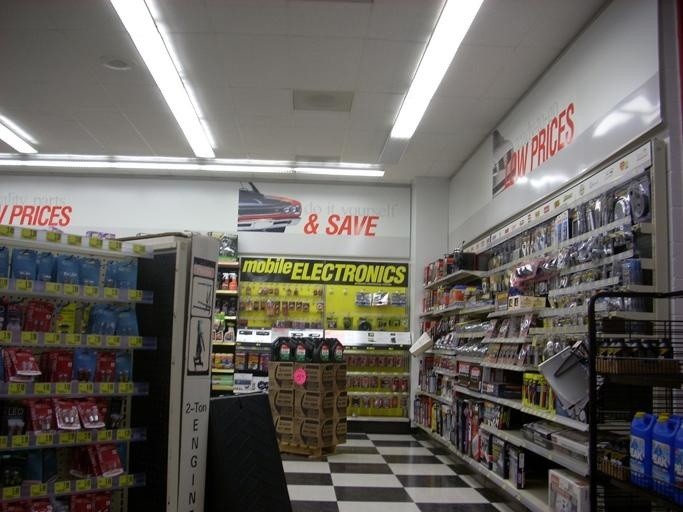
[109,0,215,158]
[0,122,39,155]
[389,0,486,141]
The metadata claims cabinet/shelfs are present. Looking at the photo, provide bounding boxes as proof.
[268,361,347,460]
[586,290,682,511]
[1,224,239,509]
[413,172,669,511]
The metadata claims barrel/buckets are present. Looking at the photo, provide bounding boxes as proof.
[627,409,683,502]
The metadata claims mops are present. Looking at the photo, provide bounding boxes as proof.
[271,334,343,363]
